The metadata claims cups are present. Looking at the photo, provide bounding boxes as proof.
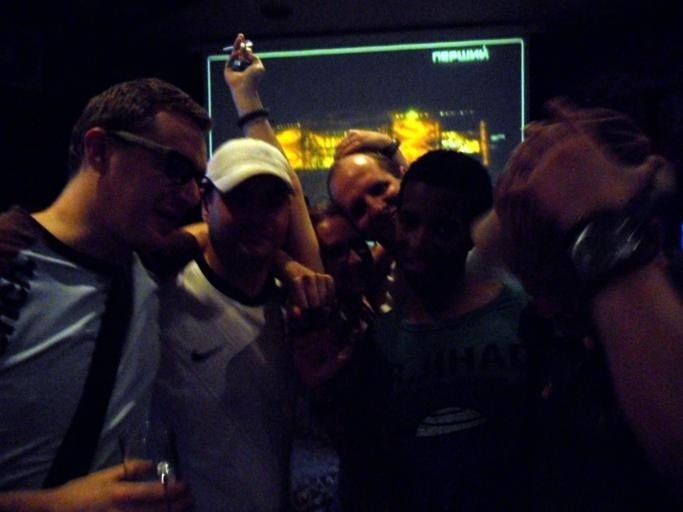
[121,434,163,482]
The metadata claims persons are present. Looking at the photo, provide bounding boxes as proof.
[0,32,683,512]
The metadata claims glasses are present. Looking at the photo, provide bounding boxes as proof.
[113,129,209,187]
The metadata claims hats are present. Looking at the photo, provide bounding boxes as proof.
[205,137,297,198]
